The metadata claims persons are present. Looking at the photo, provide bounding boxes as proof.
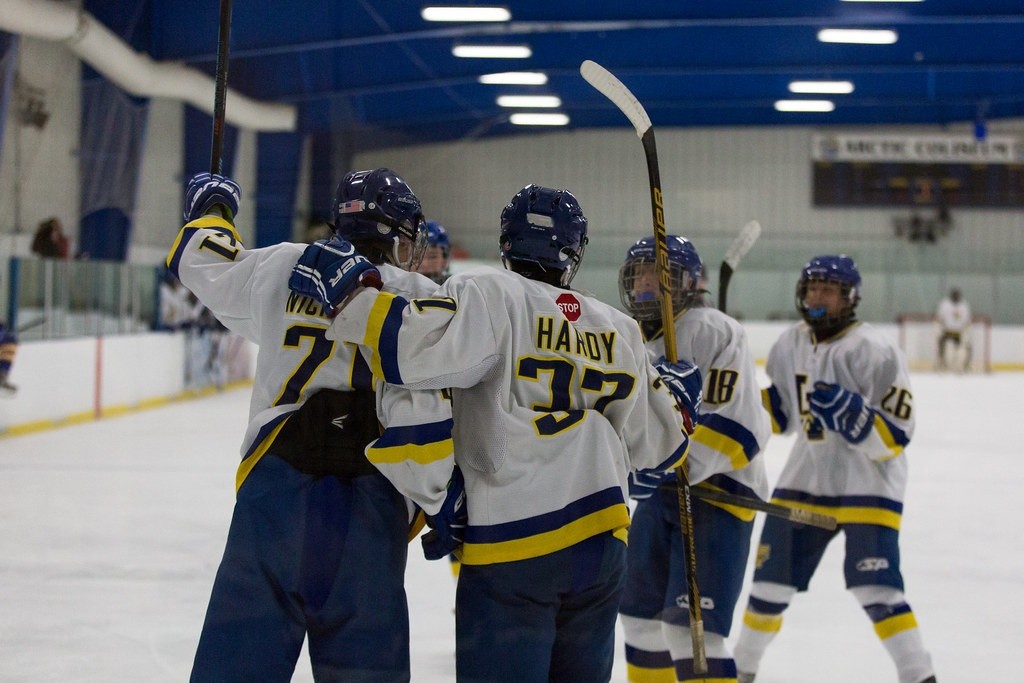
[616,234,768,683]
[411,220,452,287]
[32,219,68,261]
[937,288,971,369]
[165,167,466,683]
[731,254,938,683]
[286,182,691,683]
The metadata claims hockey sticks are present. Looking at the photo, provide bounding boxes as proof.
[577,56,712,678]
[626,466,839,537]
[715,215,765,314]
[207,1,238,177]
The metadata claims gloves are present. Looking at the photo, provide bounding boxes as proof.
[287,234,384,319]
[652,355,704,426]
[628,468,676,500]
[421,465,468,560]
[806,380,875,444]
[183,171,242,223]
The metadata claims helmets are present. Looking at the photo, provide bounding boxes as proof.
[416,221,451,259]
[499,183,588,290]
[330,167,431,272]
[618,234,705,321]
[795,254,863,330]
